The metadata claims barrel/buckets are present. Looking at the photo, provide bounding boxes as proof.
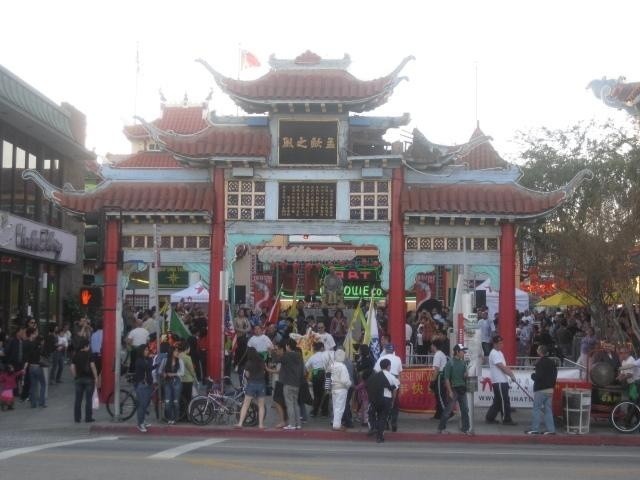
[562,387,593,434]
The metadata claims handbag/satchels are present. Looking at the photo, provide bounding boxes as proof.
[233,365,238,372]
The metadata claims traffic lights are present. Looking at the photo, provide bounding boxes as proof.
[77,286,103,309]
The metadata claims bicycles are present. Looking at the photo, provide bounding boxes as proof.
[188,373,266,427]
[105,364,188,423]
[609,400,640,434]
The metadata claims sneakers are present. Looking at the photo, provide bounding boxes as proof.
[283,425,301,430]
[430,413,474,436]
[486,419,555,435]
[137,420,152,433]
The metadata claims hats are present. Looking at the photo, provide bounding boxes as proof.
[493,335,503,343]
[383,343,393,351]
[453,343,468,351]
